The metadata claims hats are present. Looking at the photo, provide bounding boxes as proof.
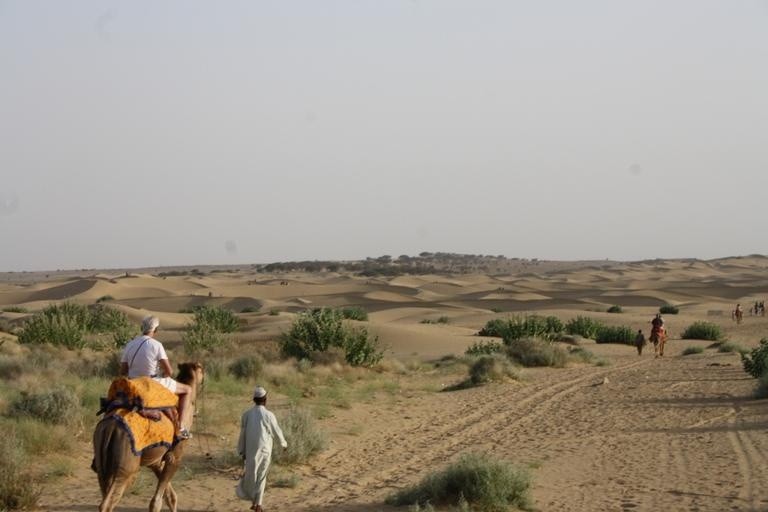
[254,386,267,398]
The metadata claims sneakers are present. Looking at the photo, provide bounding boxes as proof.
[177,428,193,440]
[250,505,263,512]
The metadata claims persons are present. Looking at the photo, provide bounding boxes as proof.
[732,299,765,324]
[118,314,195,440]
[235,386,289,512]
[651,313,664,336]
[634,329,647,355]
